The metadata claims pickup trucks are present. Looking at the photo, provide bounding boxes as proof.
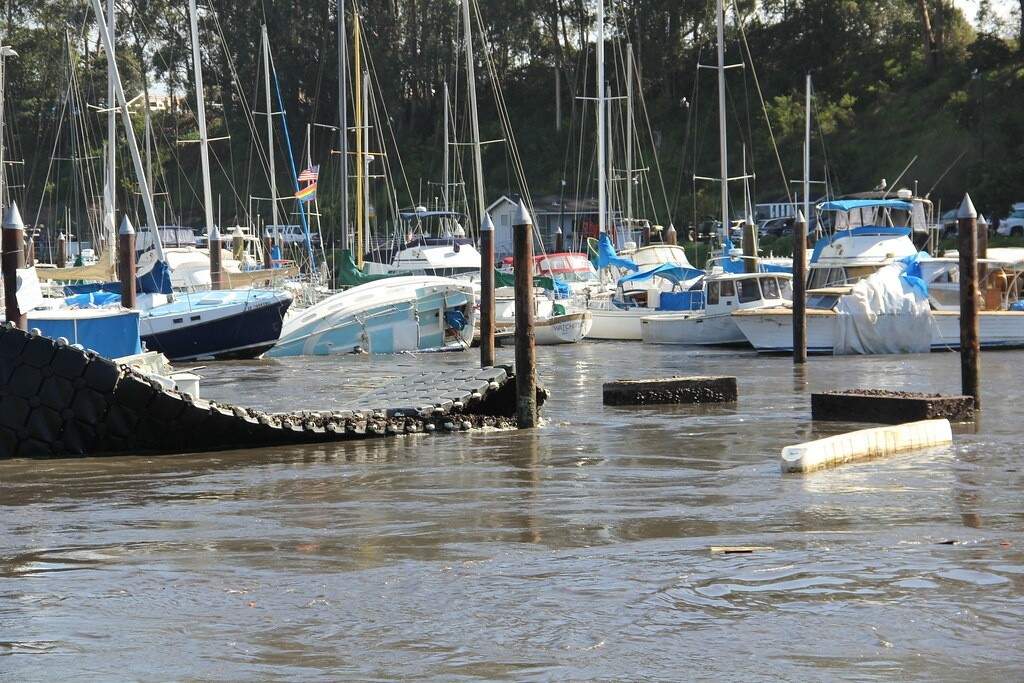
[266,225,316,244]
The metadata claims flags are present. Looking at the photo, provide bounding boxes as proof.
[294,166,318,203]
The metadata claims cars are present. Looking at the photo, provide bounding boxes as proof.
[734,216,793,248]
[697,222,725,243]
[929,208,961,239]
[986,201,1024,239]
[785,215,836,239]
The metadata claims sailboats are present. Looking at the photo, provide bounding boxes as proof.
[1,0,1024,364]
